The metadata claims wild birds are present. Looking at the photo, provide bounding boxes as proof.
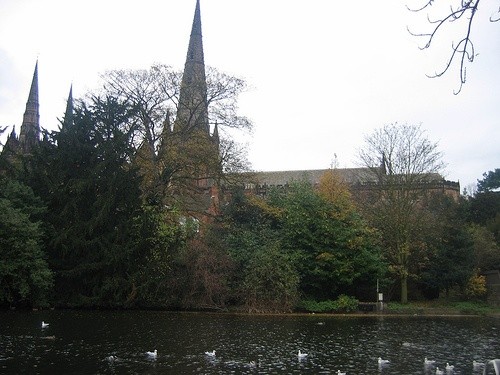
[472,360,484,367]
[249,358,262,368]
[41,320,50,329]
[424,357,436,365]
[445,362,455,371]
[144,349,158,359]
[298,349,308,358]
[205,349,217,357]
[377,357,390,364]
[337,370,346,375]
[435,366,444,375]
[104,355,118,362]
